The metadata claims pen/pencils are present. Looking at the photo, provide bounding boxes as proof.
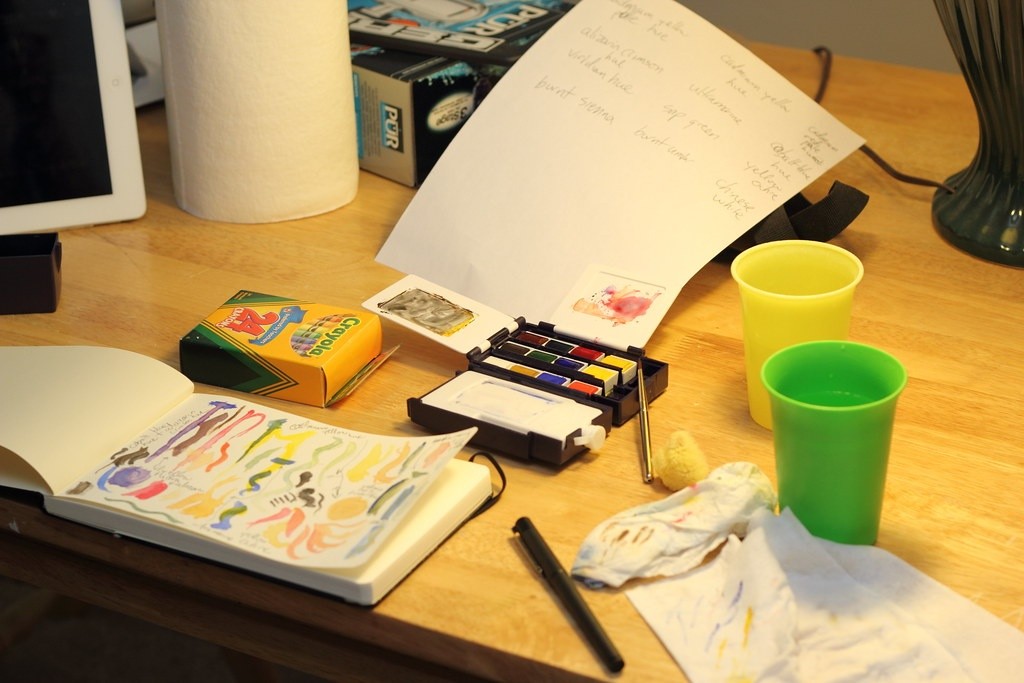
[510,513,626,674]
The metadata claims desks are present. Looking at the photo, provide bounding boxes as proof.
[0,42,1024,683]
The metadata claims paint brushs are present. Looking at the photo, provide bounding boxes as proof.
[636,355,656,484]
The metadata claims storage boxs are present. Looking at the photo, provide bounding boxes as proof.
[178,290,403,408]
[362,274,669,465]
[346,0,585,187]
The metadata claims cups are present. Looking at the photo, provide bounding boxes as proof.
[730,239,906,546]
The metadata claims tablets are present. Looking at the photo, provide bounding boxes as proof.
[0,0,146,234]
